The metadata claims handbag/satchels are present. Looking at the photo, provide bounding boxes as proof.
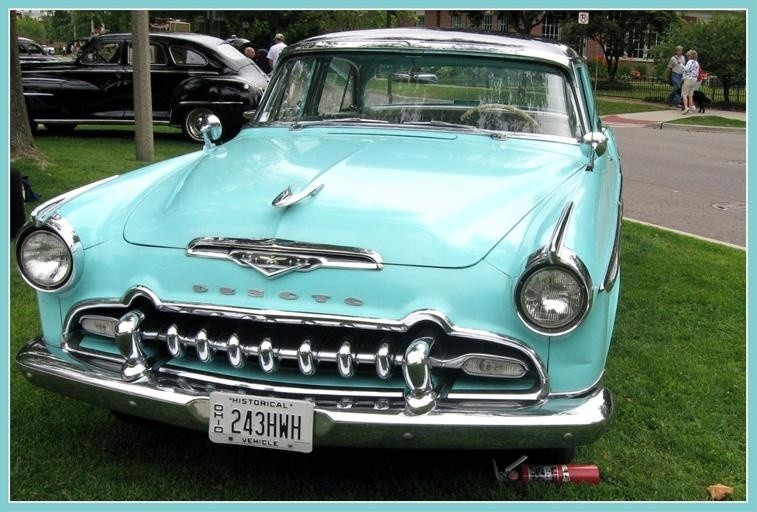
[698,64,703,81]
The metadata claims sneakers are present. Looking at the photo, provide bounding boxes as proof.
[675,104,694,115]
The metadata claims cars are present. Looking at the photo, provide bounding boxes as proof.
[13,17,626,464]
[19,29,268,147]
[15,36,59,64]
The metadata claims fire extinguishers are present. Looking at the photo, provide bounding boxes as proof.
[492,456,600,485]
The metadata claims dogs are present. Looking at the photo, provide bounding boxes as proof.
[676,87,712,113]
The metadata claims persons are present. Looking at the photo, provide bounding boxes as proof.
[265,32,289,72]
[680,49,702,117]
[242,45,269,75]
[664,43,686,108]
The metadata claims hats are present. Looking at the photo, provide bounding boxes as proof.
[273,33,285,40]
